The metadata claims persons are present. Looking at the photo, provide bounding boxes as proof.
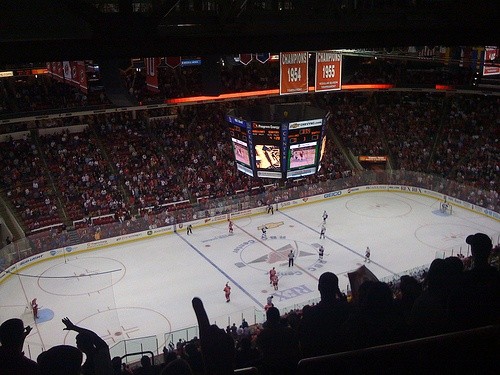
[224,283,231,302]
[0,60,500,375]
[288,250,294,267]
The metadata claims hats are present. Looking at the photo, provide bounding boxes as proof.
[466,233,493,247]
[429,256,467,296]
